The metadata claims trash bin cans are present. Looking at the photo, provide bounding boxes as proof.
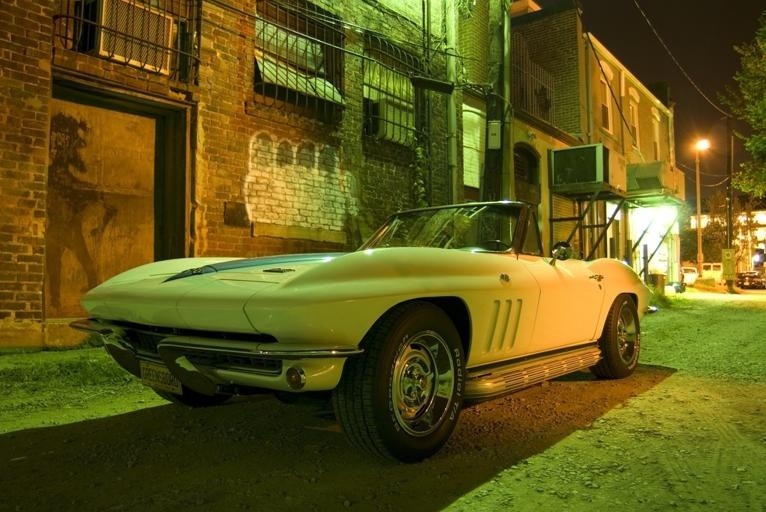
[645,274,665,298]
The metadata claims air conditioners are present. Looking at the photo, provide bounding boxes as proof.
[548,143,627,201]
[366,98,415,148]
[627,161,686,209]
[75,0,173,76]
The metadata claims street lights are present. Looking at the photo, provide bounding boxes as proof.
[693,138,711,275]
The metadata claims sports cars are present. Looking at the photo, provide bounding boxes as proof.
[69,200,657,464]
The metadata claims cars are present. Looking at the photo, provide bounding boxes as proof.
[682,263,765,291]
